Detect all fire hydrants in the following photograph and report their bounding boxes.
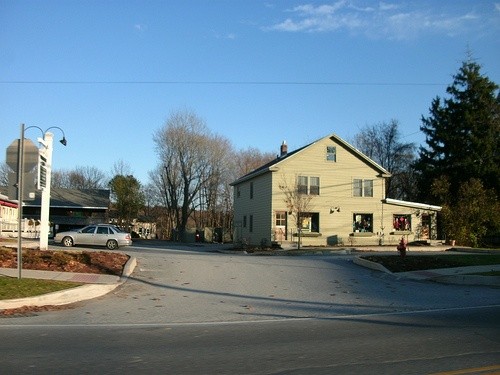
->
[397,237,406,258]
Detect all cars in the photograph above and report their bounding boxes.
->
[54,223,133,250]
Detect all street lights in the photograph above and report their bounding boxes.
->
[22,125,67,251]
